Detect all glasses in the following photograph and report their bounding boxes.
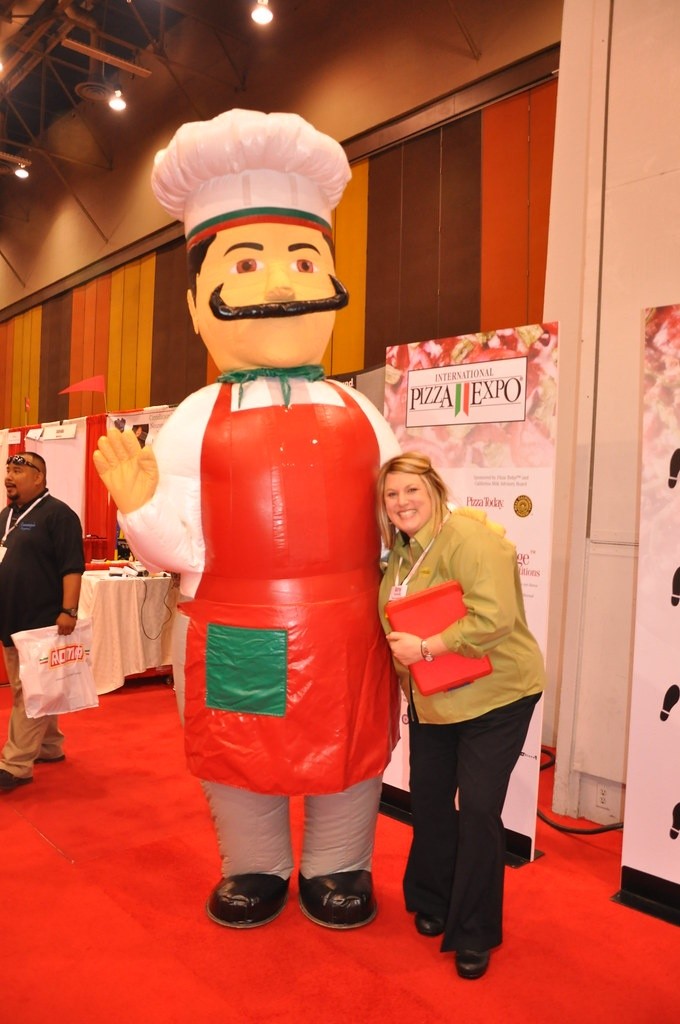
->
[6,456,41,472]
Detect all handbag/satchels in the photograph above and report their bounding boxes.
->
[10,620,99,719]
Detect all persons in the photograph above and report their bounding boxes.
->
[394,589,400,596]
[379,452,546,979]
[94,107,506,926]
[0,452,86,795]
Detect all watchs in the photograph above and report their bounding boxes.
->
[421,640,433,661]
[62,609,77,617]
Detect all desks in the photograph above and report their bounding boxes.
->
[76,570,181,697]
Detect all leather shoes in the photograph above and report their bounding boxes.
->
[456,950,489,978]
[415,914,444,936]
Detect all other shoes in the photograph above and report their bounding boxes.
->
[36,754,65,763]
[0,769,33,790]
